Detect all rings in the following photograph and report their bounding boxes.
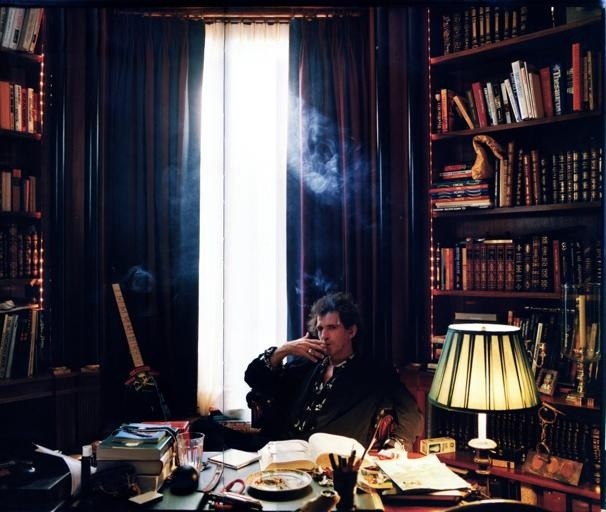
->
[309,348,314,355]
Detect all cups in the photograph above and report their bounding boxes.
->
[175,431,205,479]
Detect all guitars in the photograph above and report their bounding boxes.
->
[111,284,174,422]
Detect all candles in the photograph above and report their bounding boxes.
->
[579,295,587,348]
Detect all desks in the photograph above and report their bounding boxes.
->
[154,452,386,511]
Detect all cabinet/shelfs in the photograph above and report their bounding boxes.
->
[0,8,100,450]
[407,6,602,512]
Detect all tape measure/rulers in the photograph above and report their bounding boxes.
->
[112,283,144,369]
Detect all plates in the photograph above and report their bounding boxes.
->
[245,468,313,497]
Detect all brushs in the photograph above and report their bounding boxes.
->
[358,415,394,469]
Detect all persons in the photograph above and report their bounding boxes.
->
[244,293,423,463]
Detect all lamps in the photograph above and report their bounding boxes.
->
[428,323,540,499]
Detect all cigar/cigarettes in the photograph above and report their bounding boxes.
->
[320,344,326,348]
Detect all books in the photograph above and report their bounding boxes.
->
[255,432,369,465]
[95,418,189,493]
[376,455,472,507]
[0,3,48,379]
[209,448,257,470]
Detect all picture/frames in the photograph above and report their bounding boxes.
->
[536,369,557,398]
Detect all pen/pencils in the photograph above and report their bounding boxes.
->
[327,451,355,471]
